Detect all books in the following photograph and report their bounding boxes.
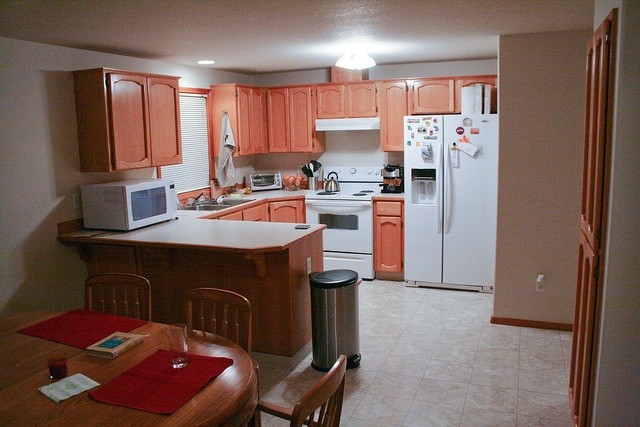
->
[84,331,145,360]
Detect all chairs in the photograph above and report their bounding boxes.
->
[81,270,153,318]
[247,353,351,427]
[182,285,253,356]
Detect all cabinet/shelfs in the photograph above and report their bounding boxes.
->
[375,200,402,272]
[74,68,152,171]
[379,80,410,152]
[313,81,376,117]
[265,86,327,155]
[209,82,252,156]
[248,84,264,156]
[147,74,182,165]
[269,200,305,223]
[413,74,499,115]
[218,202,271,224]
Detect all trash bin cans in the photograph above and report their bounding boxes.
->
[308,268,361,371]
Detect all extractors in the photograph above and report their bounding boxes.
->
[315,117,384,134]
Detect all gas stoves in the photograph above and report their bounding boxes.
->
[305,183,379,201]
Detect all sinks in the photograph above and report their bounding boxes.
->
[181,205,230,211]
[205,199,255,206]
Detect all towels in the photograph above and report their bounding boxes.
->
[88,347,236,418]
[17,308,147,351]
[215,111,236,189]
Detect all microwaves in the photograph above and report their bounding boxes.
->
[81,176,179,232]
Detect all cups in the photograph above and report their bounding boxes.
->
[164,323,190,369]
[46,348,70,379]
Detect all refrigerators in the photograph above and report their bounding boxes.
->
[402,114,499,294]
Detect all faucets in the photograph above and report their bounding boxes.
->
[192,192,204,206]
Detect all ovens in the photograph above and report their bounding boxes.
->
[244,171,283,192]
[305,199,375,281]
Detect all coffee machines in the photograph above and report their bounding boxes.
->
[378,163,404,195]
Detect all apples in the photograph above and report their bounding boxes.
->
[282,173,303,191]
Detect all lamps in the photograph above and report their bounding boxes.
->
[334,51,377,70]
